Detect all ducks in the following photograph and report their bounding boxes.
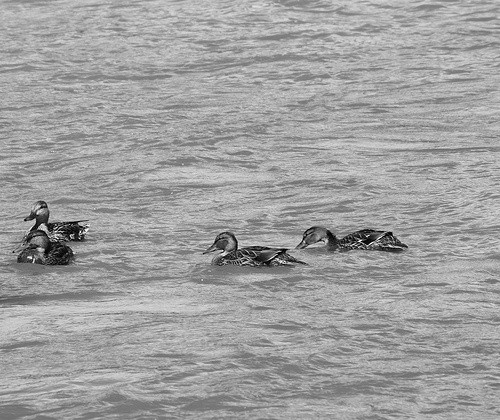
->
[202,231,311,271]
[12,201,90,265]
[294,225,409,254]
